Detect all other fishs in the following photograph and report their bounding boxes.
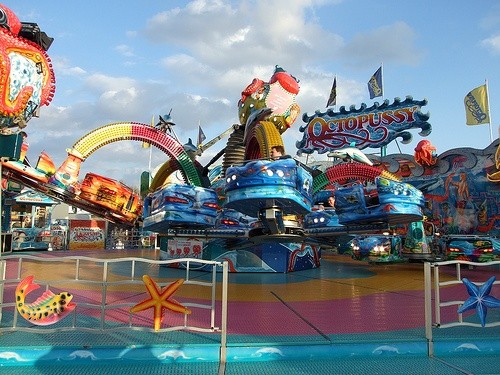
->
[16,274,77,328]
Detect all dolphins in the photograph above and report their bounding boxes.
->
[235,107,274,148]
[328,141,383,167]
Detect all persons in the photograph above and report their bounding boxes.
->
[327,196,335,207]
[271,146,284,161]
[111,227,123,235]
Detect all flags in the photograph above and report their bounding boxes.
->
[326,77,336,107]
[464,84,490,125]
[368,67,382,99]
